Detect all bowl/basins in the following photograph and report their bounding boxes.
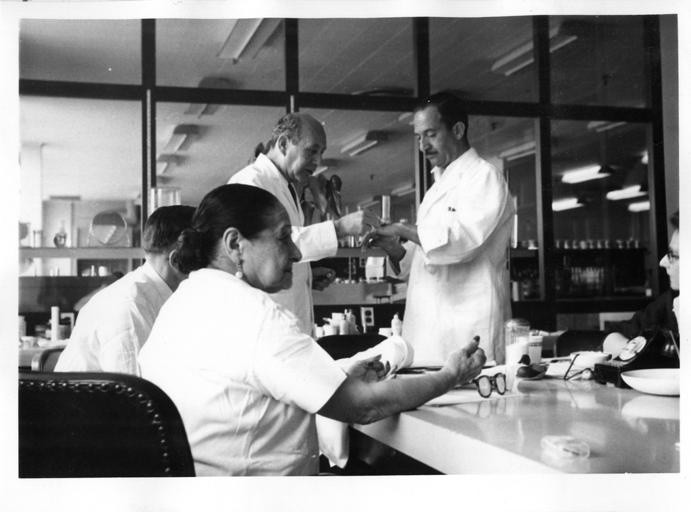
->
[571,350,606,372]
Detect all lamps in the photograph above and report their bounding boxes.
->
[339,130,387,157]
[357,141,537,211]
[490,20,579,78]
[155,20,283,184]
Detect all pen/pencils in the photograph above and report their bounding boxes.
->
[550,359,572,363]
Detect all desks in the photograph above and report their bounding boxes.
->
[347,361,680,475]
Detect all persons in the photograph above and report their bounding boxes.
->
[604,210,680,368]
[52,206,198,376]
[360,88,514,366]
[143,184,486,476]
[227,113,380,338]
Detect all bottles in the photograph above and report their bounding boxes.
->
[314,307,357,340]
[18,315,27,341]
[503,319,531,391]
[513,238,651,300]
[391,312,402,337]
[526,335,543,363]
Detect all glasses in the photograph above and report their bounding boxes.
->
[458,373,505,397]
[668,250,679,262]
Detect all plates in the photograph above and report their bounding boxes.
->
[619,367,681,396]
